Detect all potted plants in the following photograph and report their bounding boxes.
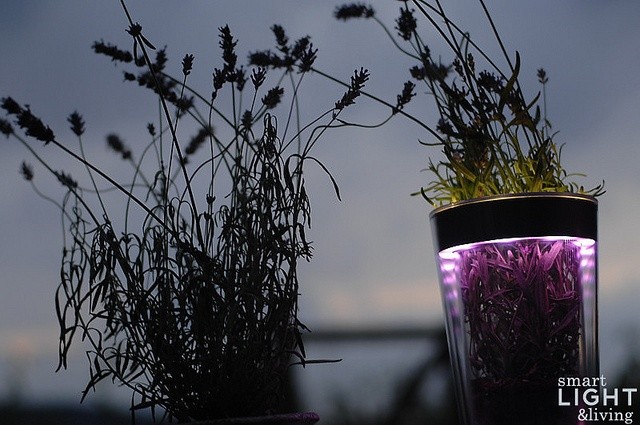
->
[247,0,606,425]
